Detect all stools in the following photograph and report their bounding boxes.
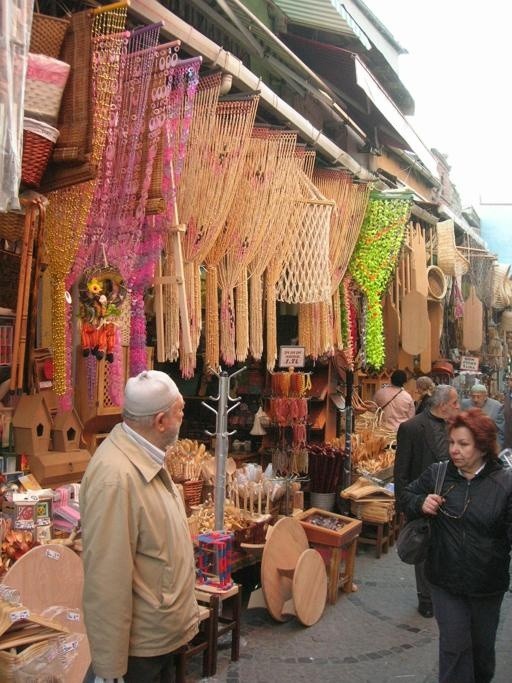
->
[171,572,244,682]
[353,509,404,559]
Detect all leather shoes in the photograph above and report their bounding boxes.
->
[418,602,433,617]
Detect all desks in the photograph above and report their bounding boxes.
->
[240,517,329,626]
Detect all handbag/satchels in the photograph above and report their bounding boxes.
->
[396,519,431,564]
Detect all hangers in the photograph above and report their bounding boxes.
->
[0,583,70,652]
[47,483,83,536]
[340,470,397,524]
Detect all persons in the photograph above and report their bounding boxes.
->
[78,369,201,683]
[375,370,512,453]
[393,385,460,618]
[399,408,512,683]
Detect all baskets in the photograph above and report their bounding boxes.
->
[184,481,205,517]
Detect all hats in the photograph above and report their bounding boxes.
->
[123,370,178,416]
[433,361,452,374]
